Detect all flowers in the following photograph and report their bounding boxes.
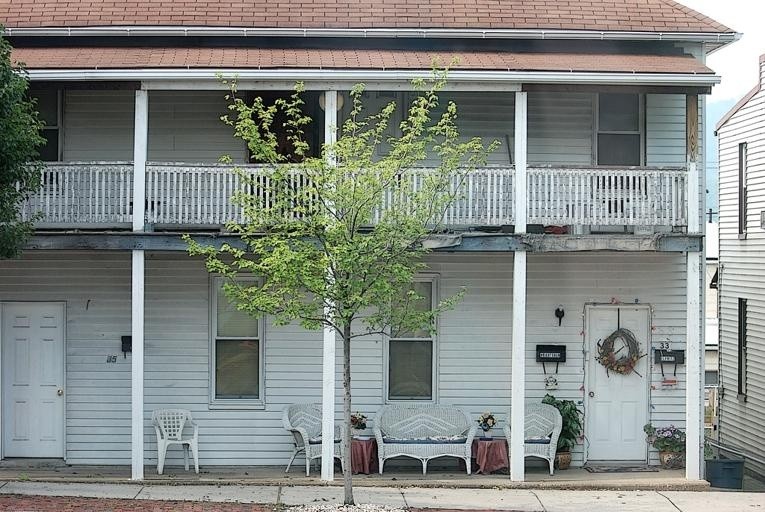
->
[642,421,684,453]
[350,411,367,429]
[594,329,647,379]
[477,411,497,429]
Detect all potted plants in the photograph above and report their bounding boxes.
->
[542,394,584,469]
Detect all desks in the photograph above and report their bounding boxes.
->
[348,438,375,474]
[474,438,508,473]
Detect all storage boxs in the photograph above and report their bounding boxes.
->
[704,457,745,489]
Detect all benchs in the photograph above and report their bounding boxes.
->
[372,403,478,474]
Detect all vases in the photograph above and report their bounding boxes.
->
[659,454,684,467]
[352,430,362,436]
[482,430,492,437]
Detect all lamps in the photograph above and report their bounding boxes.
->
[555,302,567,326]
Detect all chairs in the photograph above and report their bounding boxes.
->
[284,400,344,477]
[151,407,203,477]
[503,402,564,468]
